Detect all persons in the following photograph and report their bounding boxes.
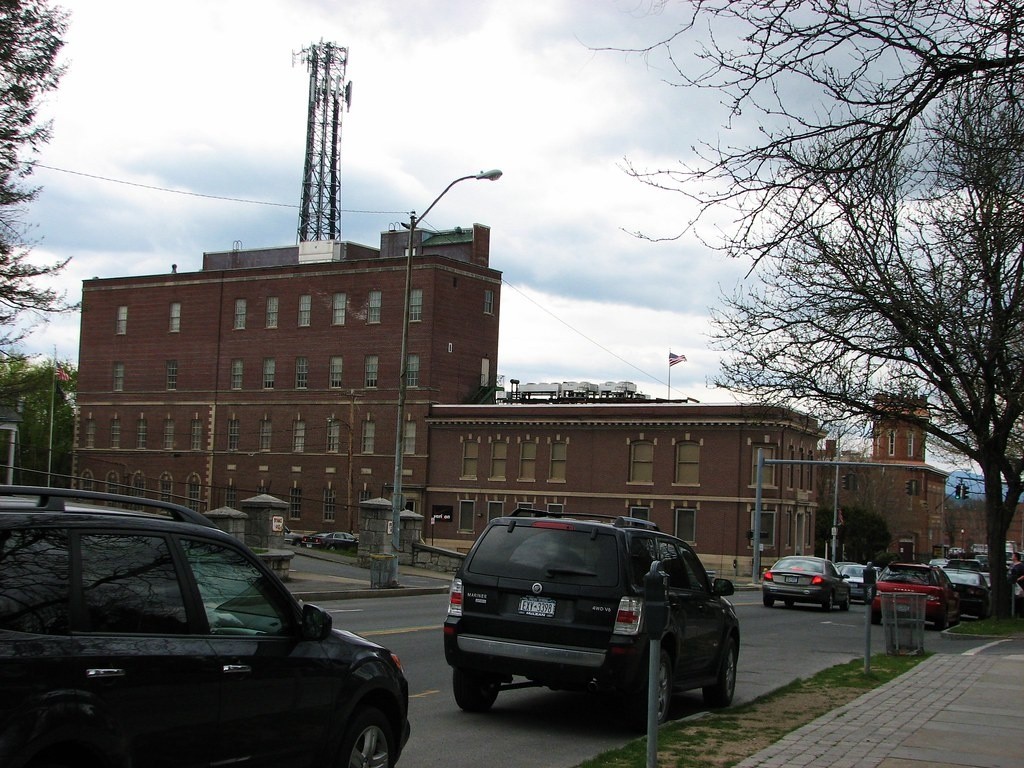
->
[863,562,876,602]
[1007,552,1024,621]
[128,555,244,628]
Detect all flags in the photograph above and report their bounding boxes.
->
[669,353,688,367]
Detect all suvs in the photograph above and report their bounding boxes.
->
[443,508,739,726]
[871,561,961,630]
[0,485,412,768]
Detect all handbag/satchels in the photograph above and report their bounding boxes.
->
[1015,582,1024,597]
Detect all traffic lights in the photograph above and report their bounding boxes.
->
[962,485,969,500]
[915,481,920,495]
[841,474,848,490]
[954,485,961,499]
[905,480,912,495]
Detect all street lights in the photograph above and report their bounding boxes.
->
[961,529,965,552]
[391,168,505,587]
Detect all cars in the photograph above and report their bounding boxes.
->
[928,540,1024,620]
[834,562,862,573]
[839,565,882,601]
[284,525,305,547]
[302,532,359,552]
[761,555,851,612]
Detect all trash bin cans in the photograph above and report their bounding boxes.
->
[877,590,927,657]
[369,552,400,590]
